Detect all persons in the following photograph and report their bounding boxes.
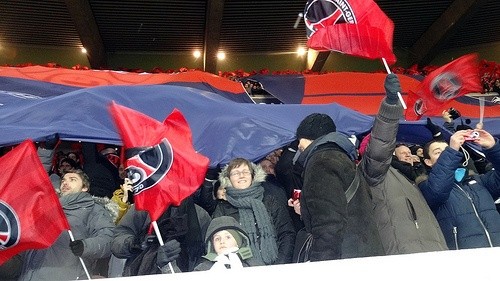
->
[0,69,500,281]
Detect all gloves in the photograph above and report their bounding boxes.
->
[131,233,157,251]
[383,74,402,105]
[155,239,182,268]
[69,239,84,257]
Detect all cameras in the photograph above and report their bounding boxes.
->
[292,189,302,204]
[464,130,480,140]
[448,107,461,120]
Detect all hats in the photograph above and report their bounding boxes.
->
[295,113,336,141]
[211,179,227,200]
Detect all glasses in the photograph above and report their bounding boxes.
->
[229,171,252,176]
[395,143,409,148]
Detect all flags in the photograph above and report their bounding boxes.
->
[403,51,482,122]
[108,99,210,234]
[0,139,71,265]
[303,0,397,67]
